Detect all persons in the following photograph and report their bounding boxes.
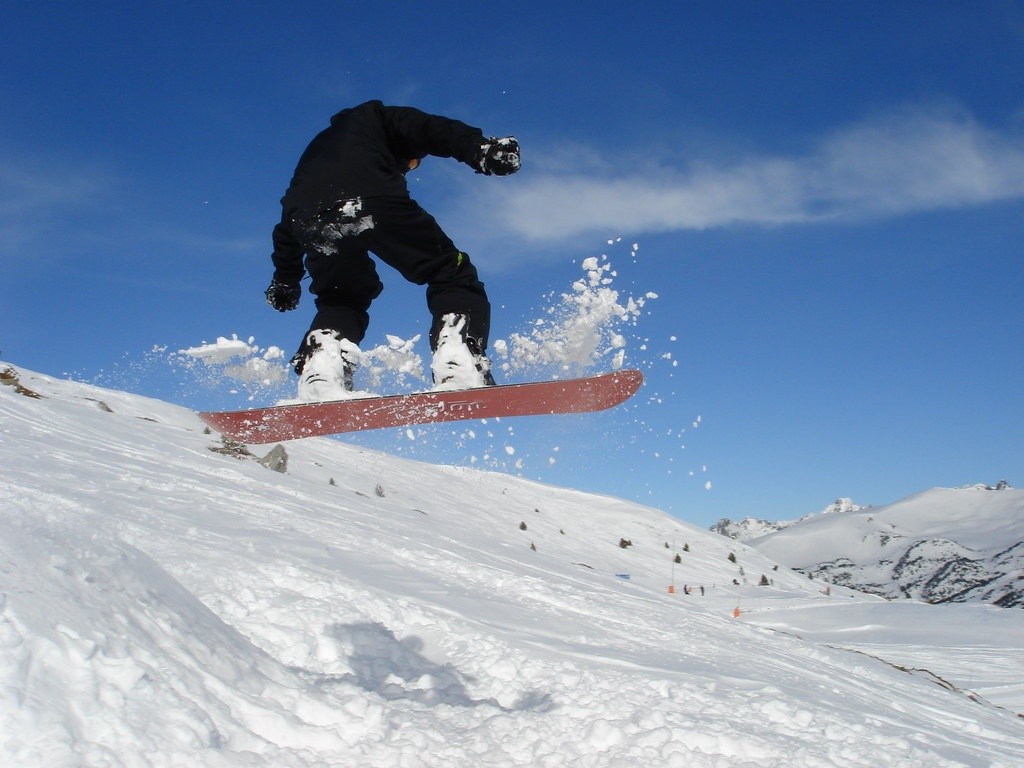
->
[265,100,521,401]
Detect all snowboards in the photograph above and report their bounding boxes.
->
[193,368,643,445]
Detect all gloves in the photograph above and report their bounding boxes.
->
[265,268,306,312]
[474,136,522,176]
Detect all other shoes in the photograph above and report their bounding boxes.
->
[431,311,497,385]
[298,328,359,395]
